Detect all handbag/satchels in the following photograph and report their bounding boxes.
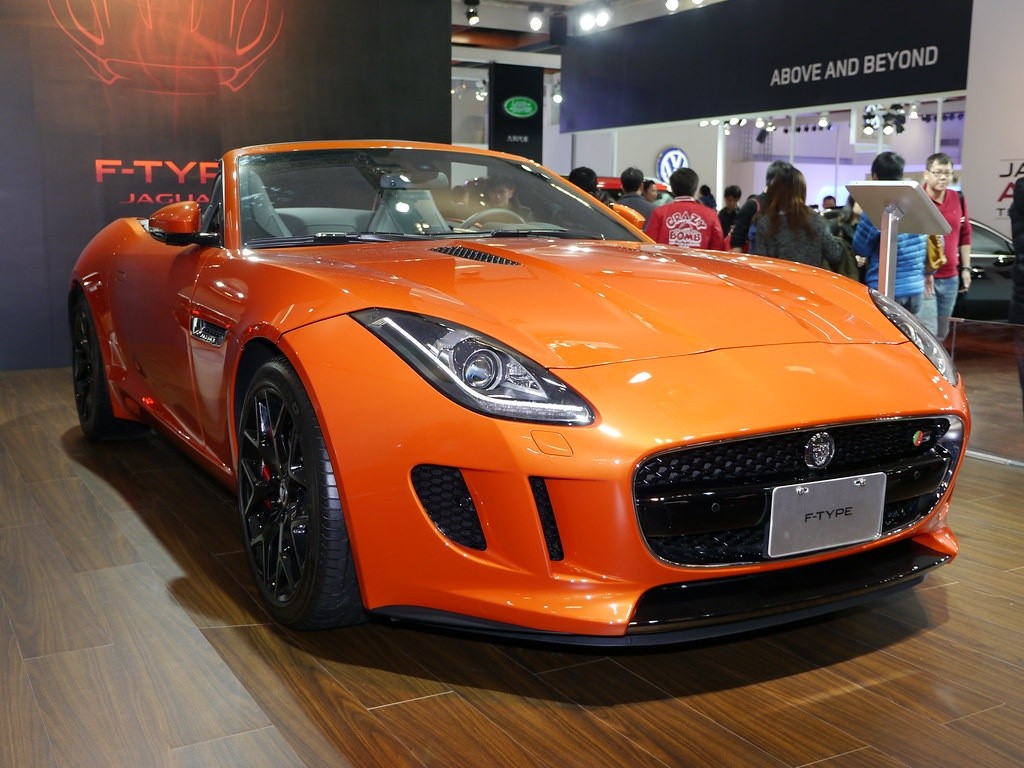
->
[723,227,751,254]
[925,232,948,275]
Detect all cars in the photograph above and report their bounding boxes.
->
[954,219,1018,332]
[561,174,677,237]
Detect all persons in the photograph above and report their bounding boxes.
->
[718,161,866,285]
[922,152,972,340]
[477,172,534,223]
[619,167,656,228]
[696,185,717,212]
[645,167,724,250]
[642,180,657,201]
[852,151,934,315]
[569,167,614,205]
[1010,176,1024,324]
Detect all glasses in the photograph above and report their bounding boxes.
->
[925,168,953,177]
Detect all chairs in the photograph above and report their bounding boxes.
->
[367,189,451,234]
[200,166,293,245]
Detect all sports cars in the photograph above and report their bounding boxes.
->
[64,138,973,648]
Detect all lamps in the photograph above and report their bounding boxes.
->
[551,90,563,104]
[766,117,776,132]
[756,129,768,144]
[907,103,920,120]
[755,117,765,128]
[783,124,833,134]
[817,112,829,127]
[465,6,479,26]
[893,115,906,134]
[862,114,876,135]
[882,113,895,135]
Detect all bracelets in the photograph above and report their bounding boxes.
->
[962,267,971,271]
